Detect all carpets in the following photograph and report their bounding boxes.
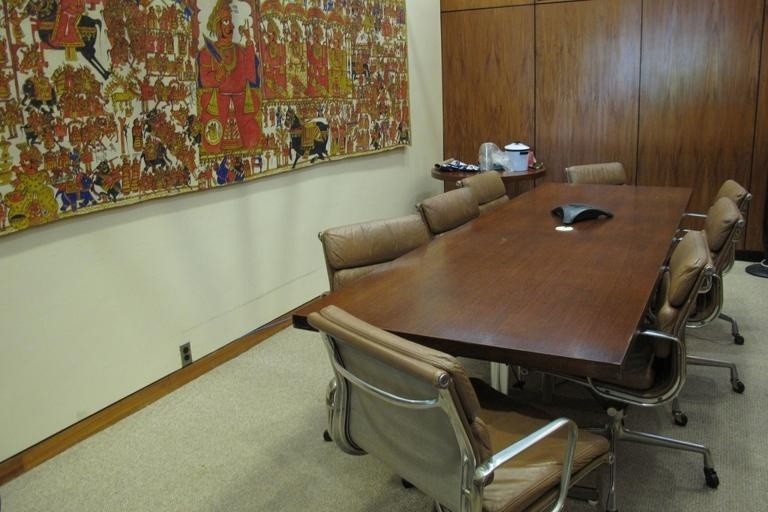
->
[0,260,768,512]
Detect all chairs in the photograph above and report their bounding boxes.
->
[536,230,720,512]
[415,187,481,238]
[565,162,626,184]
[574,197,746,427]
[456,170,510,212]
[307,304,617,512]
[317,214,434,293]
[676,180,752,345]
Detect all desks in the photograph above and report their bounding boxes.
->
[432,164,549,204]
[291,182,693,396]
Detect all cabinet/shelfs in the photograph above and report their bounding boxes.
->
[440,1,768,253]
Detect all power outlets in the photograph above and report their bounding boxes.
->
[180,342,192,368]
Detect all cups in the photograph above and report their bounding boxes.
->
[481,143,494,172]
[504,141,530,172]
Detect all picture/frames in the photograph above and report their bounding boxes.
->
[0,0,411,235]
